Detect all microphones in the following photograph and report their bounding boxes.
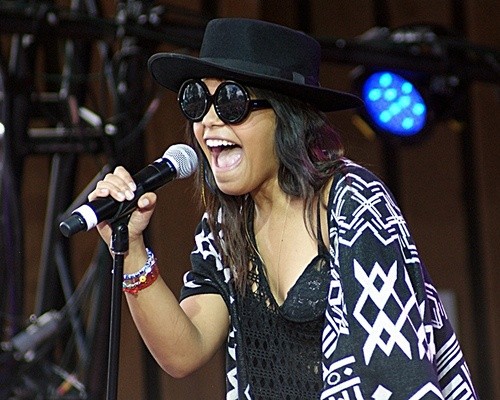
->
[58,143,199,238]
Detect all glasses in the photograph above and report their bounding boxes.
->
[177,79,273,125]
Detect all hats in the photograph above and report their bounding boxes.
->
[147,17,364,112]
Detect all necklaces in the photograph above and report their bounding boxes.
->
[243,193,291,302]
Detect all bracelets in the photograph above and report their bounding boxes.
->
[112,249,160,293]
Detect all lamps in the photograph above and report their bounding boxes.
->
[351,33,460,138]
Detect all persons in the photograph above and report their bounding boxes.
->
[88,20,478,399]
